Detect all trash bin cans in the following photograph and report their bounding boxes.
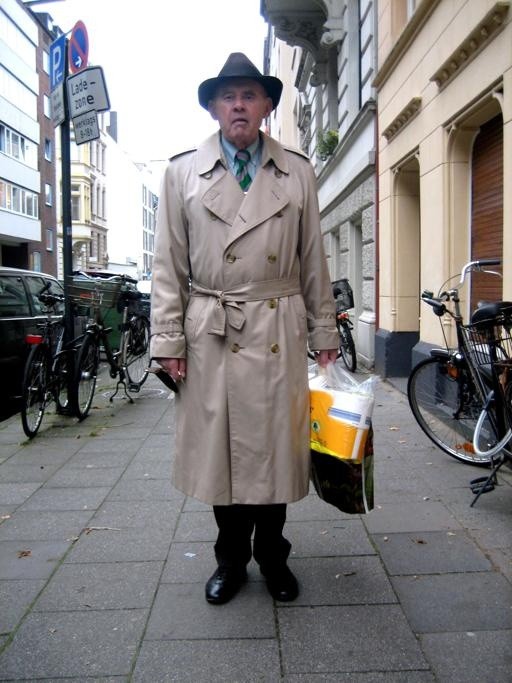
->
[60,279,125,352]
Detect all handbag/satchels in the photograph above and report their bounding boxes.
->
[298,358,377,514]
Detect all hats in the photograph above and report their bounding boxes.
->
[197,51,282,113]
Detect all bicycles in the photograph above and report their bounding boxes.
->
[406,260,512,508]
[308,278,357,372]
[17,271,152,436]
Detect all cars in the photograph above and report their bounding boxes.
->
[0,266,100,414]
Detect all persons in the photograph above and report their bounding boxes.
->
[149,51,343,604]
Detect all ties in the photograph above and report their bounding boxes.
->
[232,151,253,192]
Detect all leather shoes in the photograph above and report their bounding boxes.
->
[258,562,301,602]
[204,564,250,606]
